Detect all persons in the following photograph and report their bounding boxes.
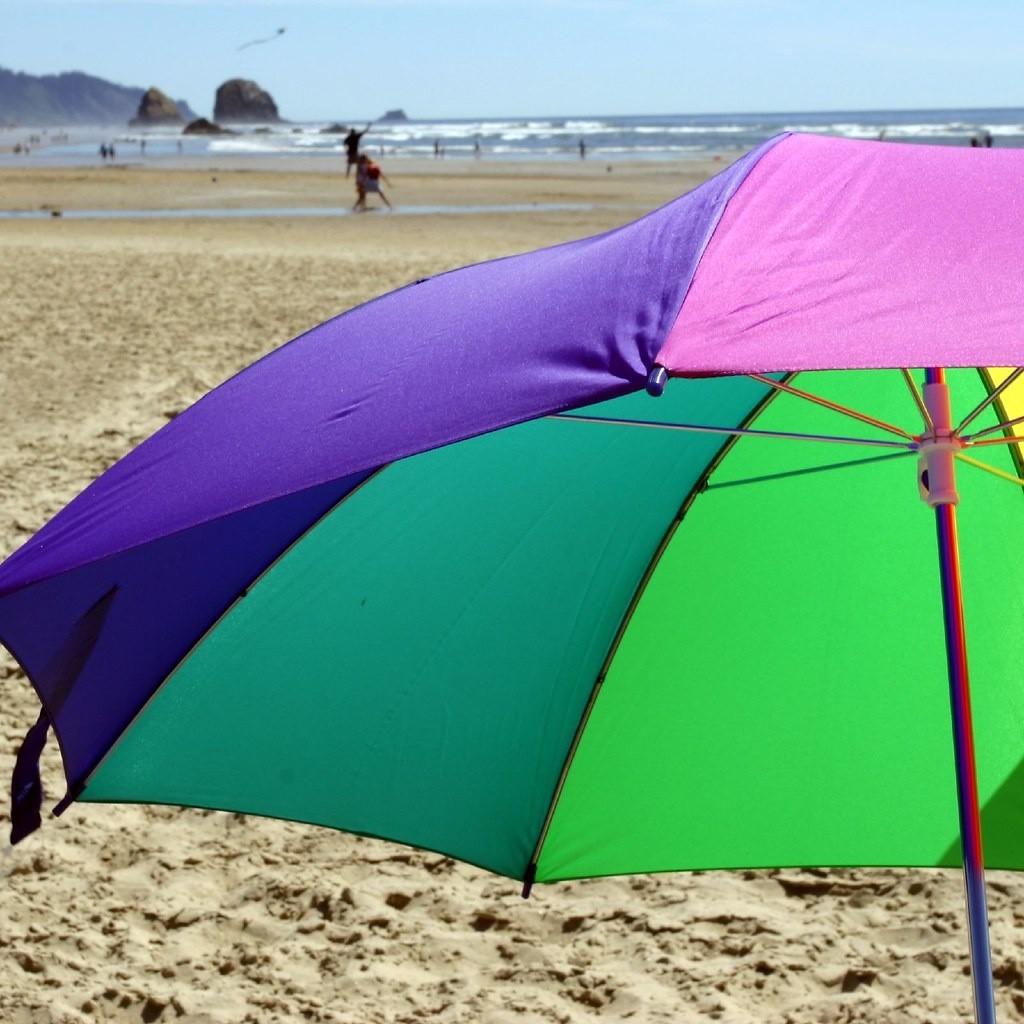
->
[579,139,585,159]
[99,144,116,159]
[344,123,394,210]
[434,138,438,152]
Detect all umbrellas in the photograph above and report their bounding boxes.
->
[0,131,1024,1023]
